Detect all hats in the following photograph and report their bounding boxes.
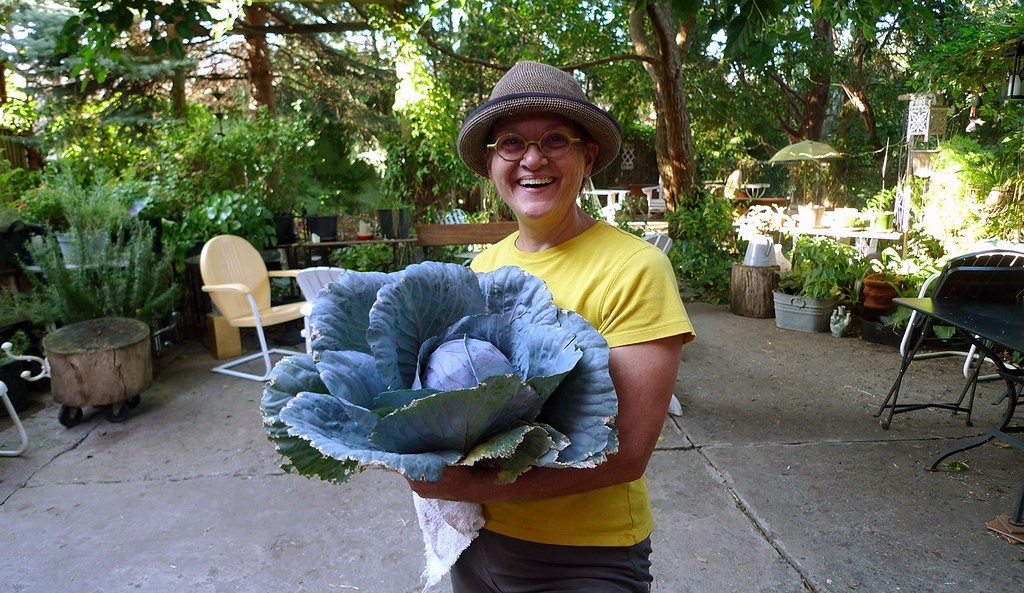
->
[458,61,623,180]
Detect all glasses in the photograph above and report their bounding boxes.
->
[485,130,582,162]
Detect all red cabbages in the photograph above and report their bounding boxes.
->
[259,257,622,486]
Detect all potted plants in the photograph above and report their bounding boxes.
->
[870,208,894,232]
[798,201,825,227]
[834,205,858,226]
[180,189,277,358]
[772,235,857,332]
[851,244,908,308]
[356,211,374,239]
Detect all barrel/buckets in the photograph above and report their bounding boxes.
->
[744,234,778,268]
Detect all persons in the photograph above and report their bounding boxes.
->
[445,56,701,593]
[723,158,749,199]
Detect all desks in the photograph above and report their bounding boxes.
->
[278,236,417,297]
[741,181,770,198]
[892,297,1024,471]
[43,318,155,427]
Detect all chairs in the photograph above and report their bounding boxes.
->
[705,167,743,198]
[582,176,631,221]
[642,175,667,219]
[200,233,307,381]
[0,380,29,456]
[644,232,684,416]
[871,248,1024,429]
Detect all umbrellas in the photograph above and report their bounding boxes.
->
[767,138,843,205]
